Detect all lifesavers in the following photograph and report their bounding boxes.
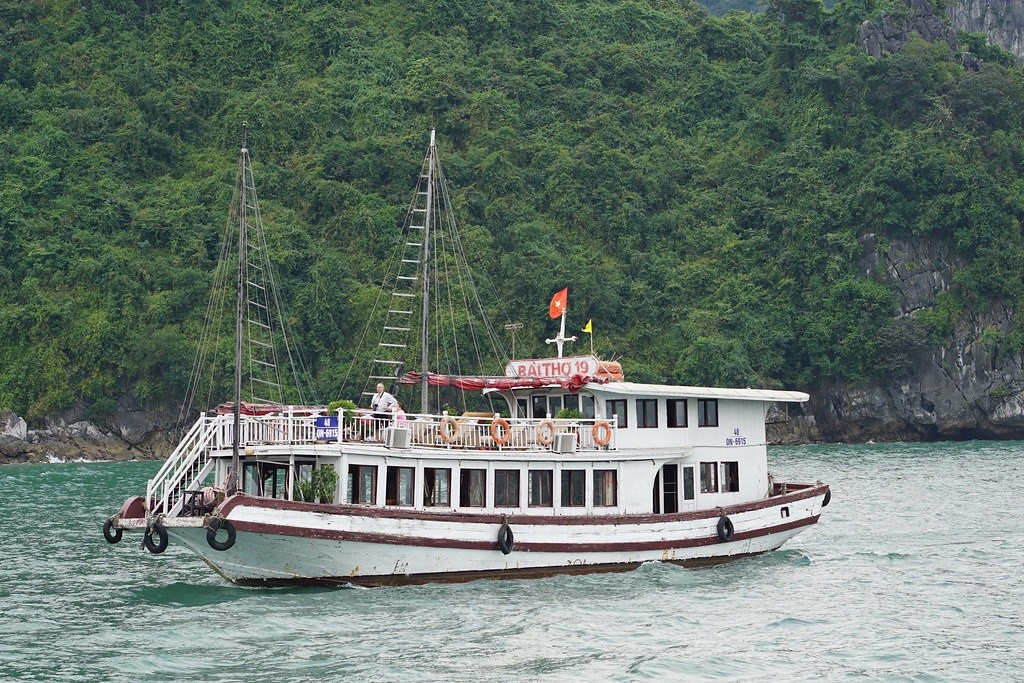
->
[206,517,237,552]
[821,486,832,507]
[536,419,557,446]
[716,516,735,542]
[592,419,612,446]
[490,417,510,444]
[144,524,169,554]
[101,517,123,545]
[497,523,514,556]
[439,416,460,444]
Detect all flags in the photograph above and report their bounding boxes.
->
[549,286,567,319]
[581,319,592,334]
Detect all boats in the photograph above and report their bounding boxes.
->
[103,120,834,592]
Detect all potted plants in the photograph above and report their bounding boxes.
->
[328,399,359,441]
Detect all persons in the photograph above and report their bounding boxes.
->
[371,383,406,441]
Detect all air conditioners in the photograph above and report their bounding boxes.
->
[385,426,411,448]
[553,433,578,454]
